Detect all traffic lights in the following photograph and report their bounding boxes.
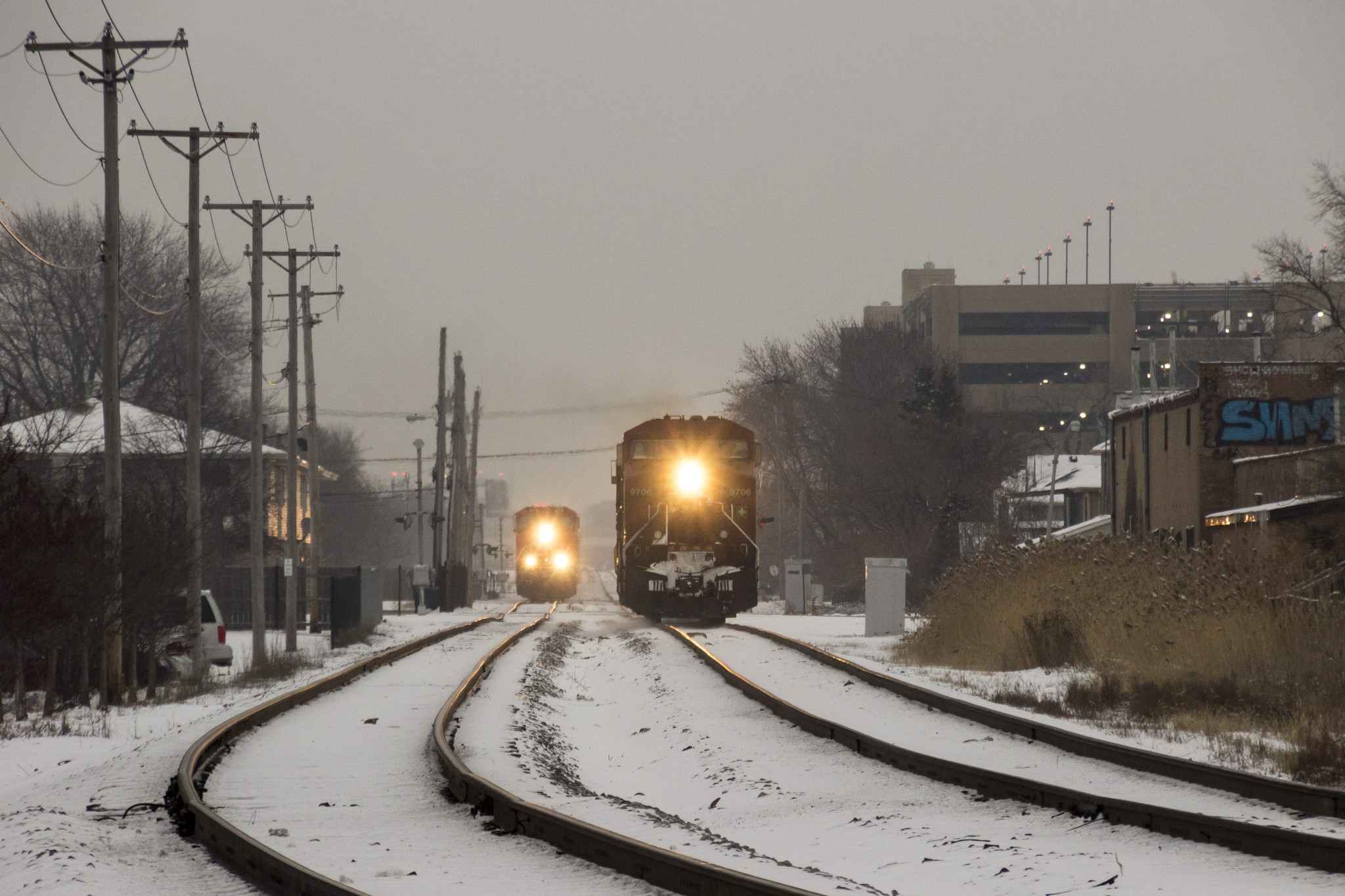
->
[394,513,412,531]
[430,511,445,530]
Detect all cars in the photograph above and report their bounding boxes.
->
[134,587,234,688]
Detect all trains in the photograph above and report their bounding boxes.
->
[512,505,582,603]
[611,414,762,628]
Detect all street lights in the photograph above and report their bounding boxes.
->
[406,414,460,564]
